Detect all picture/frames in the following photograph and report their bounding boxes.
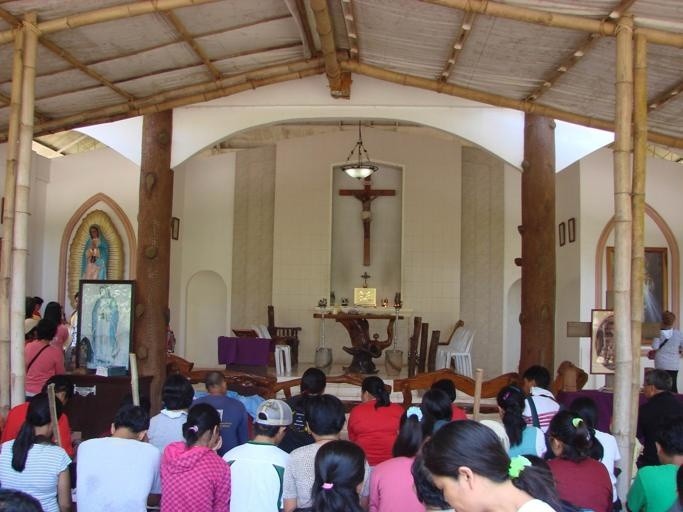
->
[605,247,669,339]
[75,279,137,374]
[589,308,617,375]
[172,216,181,242]
[568,217,576,244]
[558,221,566,246]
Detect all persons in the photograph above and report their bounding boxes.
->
[150,377,222,458]
[421,422,559,512]
[349,380,404,464]
[289,368,326,449]
[222,399,290,512]
[418,390,451,442]
[520,366,559,428]
[624,418,683,512]
[161,404,231,511]
[636,372,683,444]
[1,376,72,461]
[293,440,370,511]
[567,398,624,511]
[91,286,119,371]
[0,489,44,512]
[485,387,548,460]
[432,379,468,422]
[509,448,593,512]
[79,224,109,278]
[651,312,681,392]
[37,302,68,350]
[25,319,64,396]
[76,407,161,511]
[192,372,248,451]
[25,297,40,334]
[282,395,375,511]
[0,395,73,512]
[539,413,612,511]
[370,406,440,511]
[65,294,79,369]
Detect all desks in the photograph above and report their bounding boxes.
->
[313,308,404,376]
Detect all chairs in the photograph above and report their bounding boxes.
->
[436,321,476,376]
[252,299,301,374]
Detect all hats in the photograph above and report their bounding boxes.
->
[253,397,293,427]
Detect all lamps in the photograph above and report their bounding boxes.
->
[342,118,380,180]
